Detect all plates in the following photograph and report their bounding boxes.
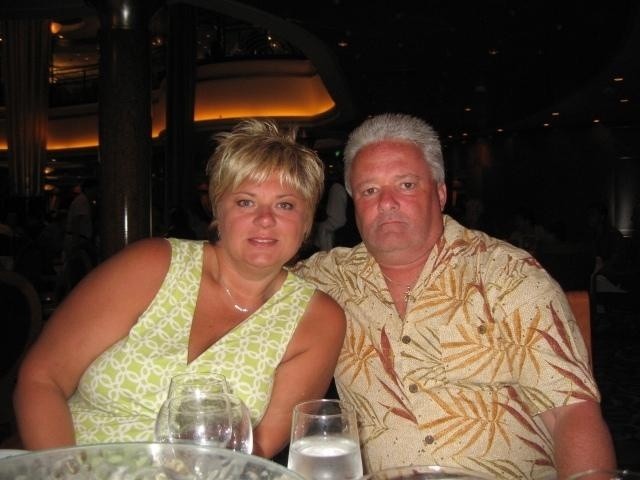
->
[0,442,304,480]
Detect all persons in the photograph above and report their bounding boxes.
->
[286,114,619,480]
[11,119,347,460]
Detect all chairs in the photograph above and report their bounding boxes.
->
[561,294,592,377]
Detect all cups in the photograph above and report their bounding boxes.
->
[151,391,253,480]
[164,371,235,480]
[286,399,363,480]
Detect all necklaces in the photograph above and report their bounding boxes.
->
[220,276,278,314]
[379,267,429,304]
